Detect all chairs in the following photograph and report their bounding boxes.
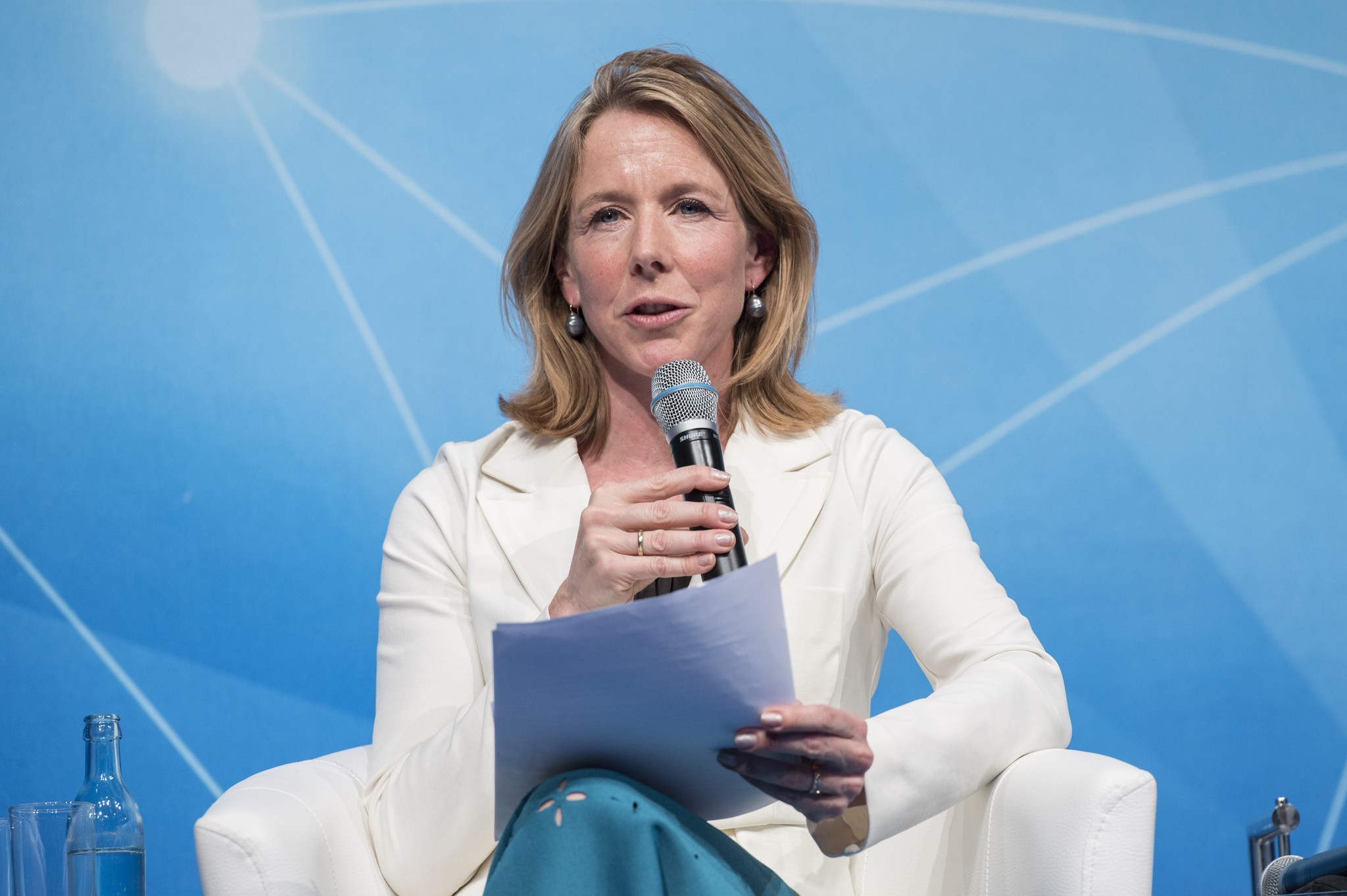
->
[196,748,1159,896]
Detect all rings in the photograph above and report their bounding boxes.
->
[638,530,643,556]
[810,763,820,794]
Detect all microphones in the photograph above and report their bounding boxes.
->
[649,360,748,582]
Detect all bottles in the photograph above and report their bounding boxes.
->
[64,713,145,896]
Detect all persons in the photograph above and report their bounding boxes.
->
[367,49,1072,896]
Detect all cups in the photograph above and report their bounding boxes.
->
[0,801,95,896]
